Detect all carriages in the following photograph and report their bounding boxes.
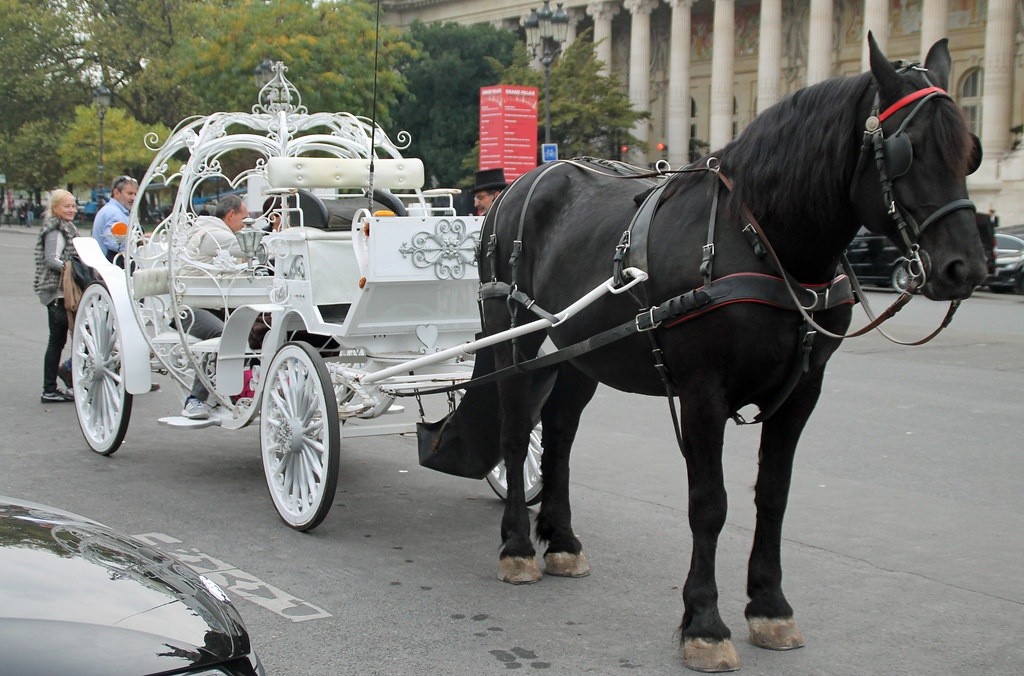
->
[57,31,1002,676]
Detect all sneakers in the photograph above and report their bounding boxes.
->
[181,397,210,419]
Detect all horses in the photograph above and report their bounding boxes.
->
[477,25,992,672]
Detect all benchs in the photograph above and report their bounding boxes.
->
[265,156,462,243]
[135,267,203,344]
[176,276,342,356]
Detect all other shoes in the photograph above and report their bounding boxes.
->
[151,383,160,391]
[65,388,93,403]
[41,389,76,402]
[57,366,73,389]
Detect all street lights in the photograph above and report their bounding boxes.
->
[92,86,111,209]
[526,1,570,164]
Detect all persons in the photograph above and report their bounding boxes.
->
[59,174,160,391]
[167,194,340,420]
[16,195,29,226]
[33,189,98,403]
[2,191,13,227]
[471,167,508,214]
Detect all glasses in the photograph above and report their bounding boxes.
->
[474,190,494,202]
[114,175,137,187]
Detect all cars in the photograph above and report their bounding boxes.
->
[0,491,269,676]
[843,220,1024,294]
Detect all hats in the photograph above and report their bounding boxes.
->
[469,168,508,195]
